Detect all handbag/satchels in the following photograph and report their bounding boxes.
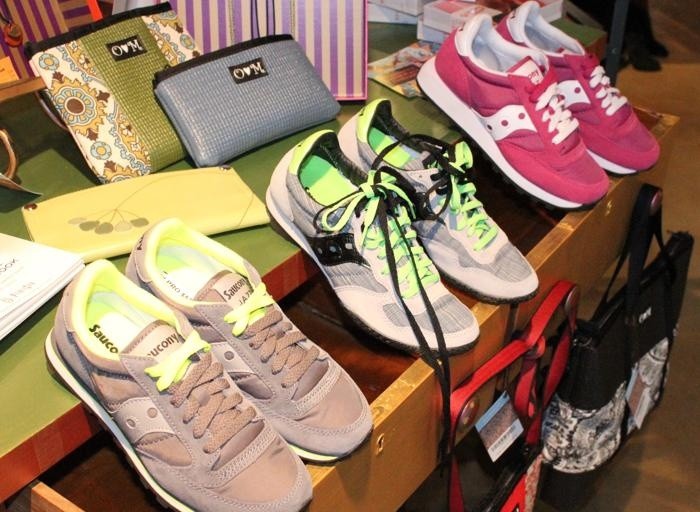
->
[542,231,692,511]
[22,165,271,264]
[23,3,205,184]
[154,34,342,168]
[478,447,543,511]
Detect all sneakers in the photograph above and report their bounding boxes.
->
[336,99,539,303]
[417,14,610,208]
[125,218,374,463]
[45,259,315,512]
[496,1,660,175]
[265,131,481,355]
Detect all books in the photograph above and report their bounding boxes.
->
[0,232,85,341]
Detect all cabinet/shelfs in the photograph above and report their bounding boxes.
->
[1,21,682,512]
